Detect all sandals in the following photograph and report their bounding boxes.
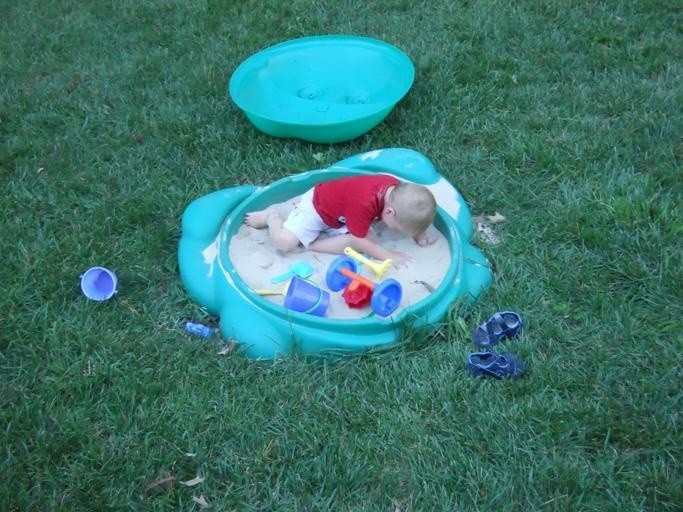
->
[467,347,525,381]
[473,223,500,250]
[473,311,522,347]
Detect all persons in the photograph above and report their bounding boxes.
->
[243,174,440,270]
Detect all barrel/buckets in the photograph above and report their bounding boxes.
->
[284,274,330,316]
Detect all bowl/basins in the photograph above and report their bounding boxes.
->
[227,34,420,140]
